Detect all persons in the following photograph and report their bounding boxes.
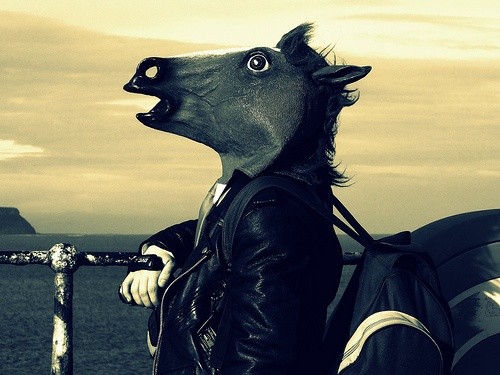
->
[115,18,373,373]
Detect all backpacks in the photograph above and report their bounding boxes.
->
[225,172,459,375]
